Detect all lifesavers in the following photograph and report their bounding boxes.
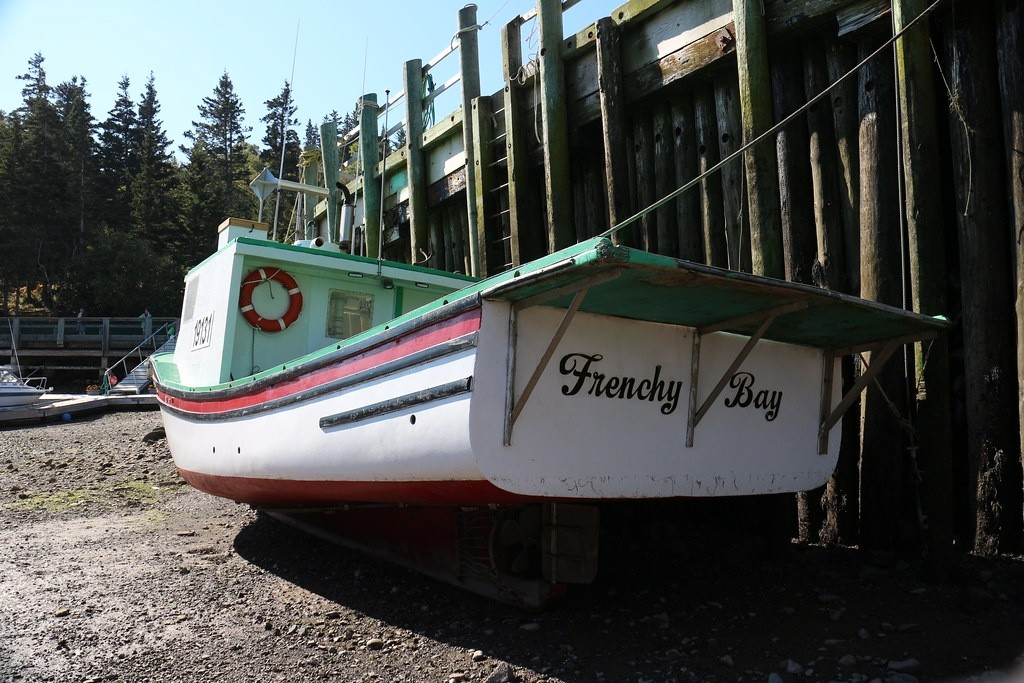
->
[237,264,305,334]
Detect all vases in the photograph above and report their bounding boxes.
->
[86,390,105,395]
[148,389,157,394]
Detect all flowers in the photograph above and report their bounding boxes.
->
[149,384,156,389]
[87,385,101,390]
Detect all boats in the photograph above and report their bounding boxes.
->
[148,218,954,609]
[0,365,49,407]
